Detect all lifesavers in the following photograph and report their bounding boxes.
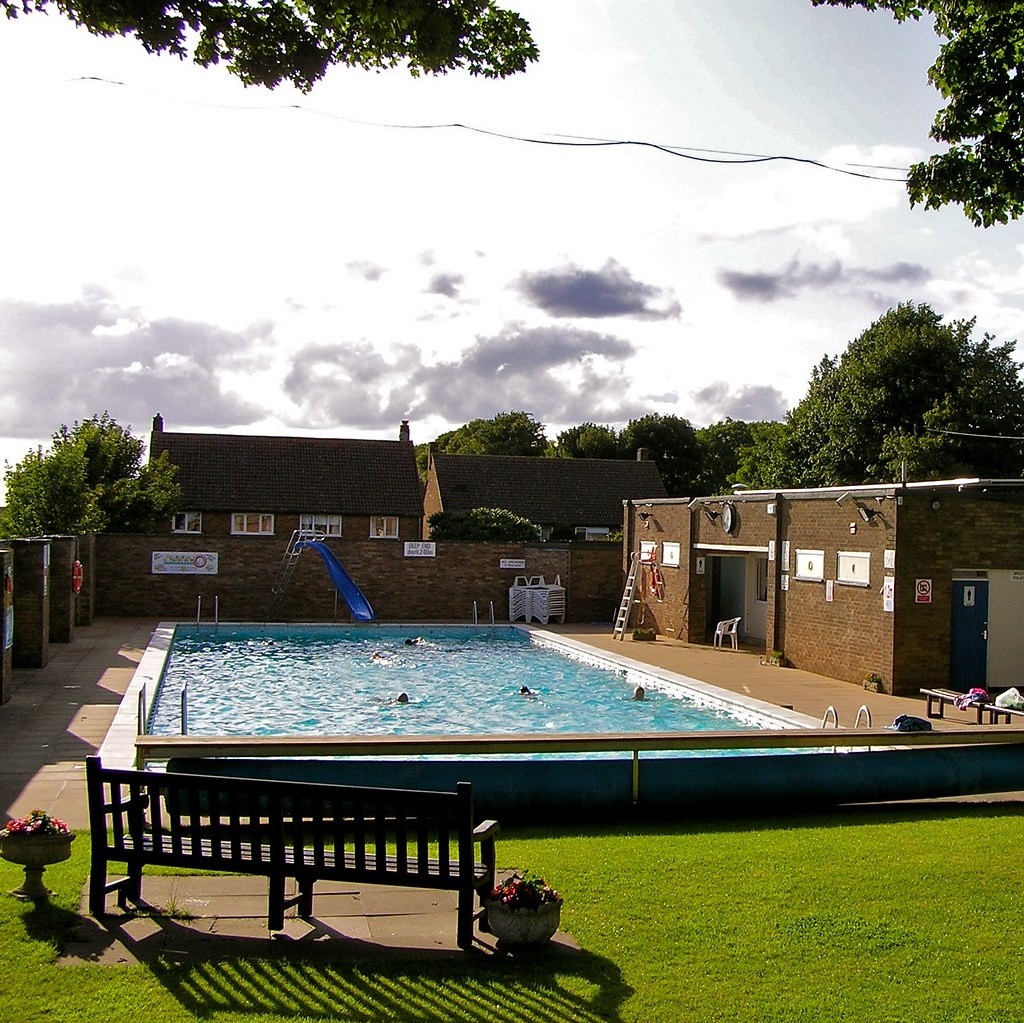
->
[73,559,84,591]
[649,567,660,596]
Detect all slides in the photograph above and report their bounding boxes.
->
[294,539,376,622]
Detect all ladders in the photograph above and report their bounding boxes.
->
[613,551,678,641]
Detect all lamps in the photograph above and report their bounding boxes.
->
[857,506,882,521]
[638,512,653,520]
[704,511,721,520]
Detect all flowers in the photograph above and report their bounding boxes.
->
[490,868,562,910]
[6,806,66,834]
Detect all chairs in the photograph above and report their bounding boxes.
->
[714,617,742,649]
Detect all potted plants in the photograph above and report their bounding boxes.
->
[863,674,882,693]
[632,628,656,641]
[770,651,789,666]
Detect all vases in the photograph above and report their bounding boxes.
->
[0,829,76,901]
[485,896,563,945]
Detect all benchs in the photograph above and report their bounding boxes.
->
[920,688,1024,725]
[88,756,497,947]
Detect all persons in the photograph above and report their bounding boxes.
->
[372,652,380,658]
[405,637,423,645]
[632,686,645,700]
[398,693,408,702]
[520,686,531,694]
